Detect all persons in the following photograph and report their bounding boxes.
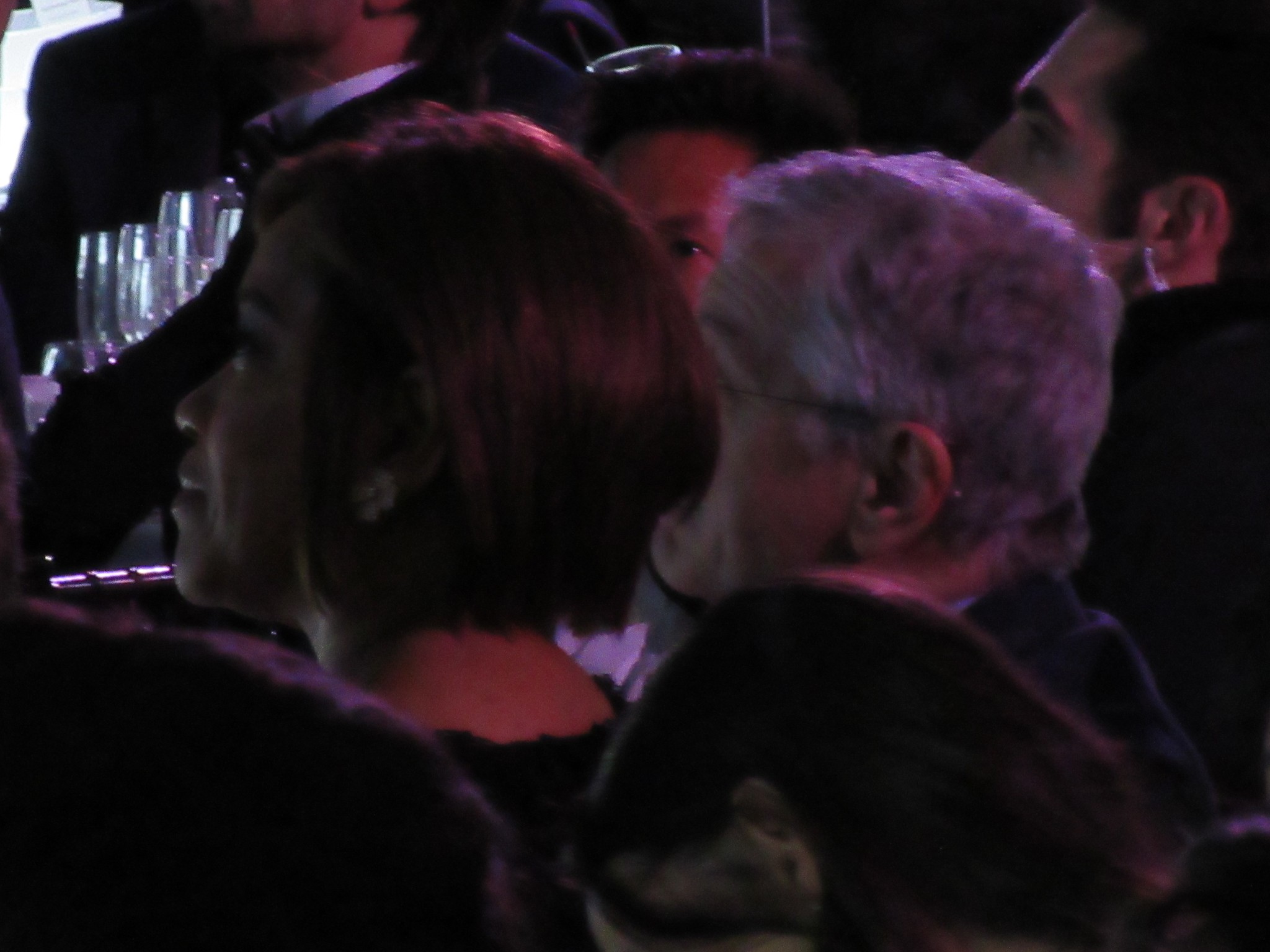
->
[955,0,1267,830]
[170,120,720,951]
[2,3,591,394]
[7,1,513,589]
[576,47,856,326]
[559,572,1269,952]
[0,579,528,951]
[659,142,1223,844]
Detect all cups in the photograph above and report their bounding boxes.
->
[45,190,247,387]
